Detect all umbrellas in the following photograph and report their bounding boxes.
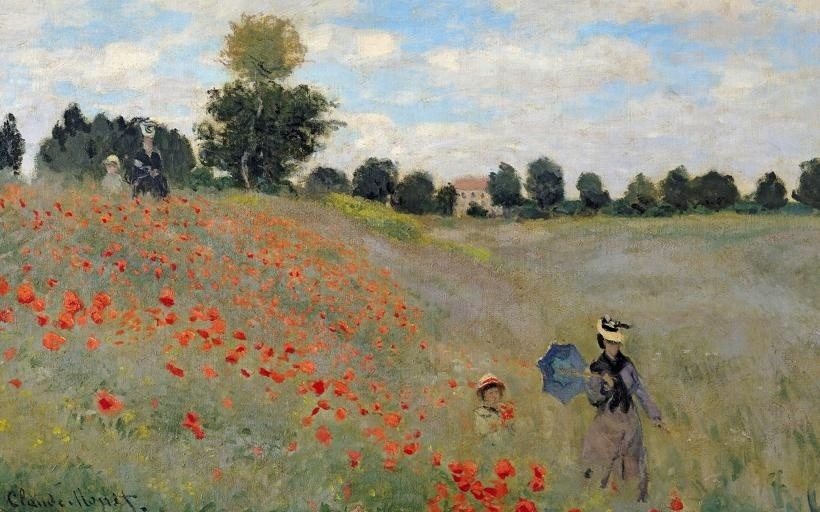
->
[535,341,617,406]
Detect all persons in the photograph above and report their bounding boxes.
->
[472,373,513,459]
[579,316,671,504]
[100,155,123,194]
[130,121,171,201]
